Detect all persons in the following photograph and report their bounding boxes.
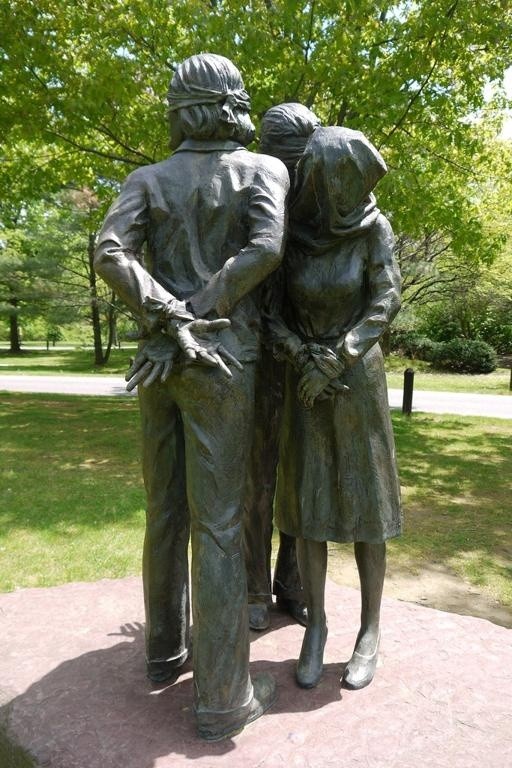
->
[93,51,289,740]
[246,102,322,630]
[254,125,403,692]
